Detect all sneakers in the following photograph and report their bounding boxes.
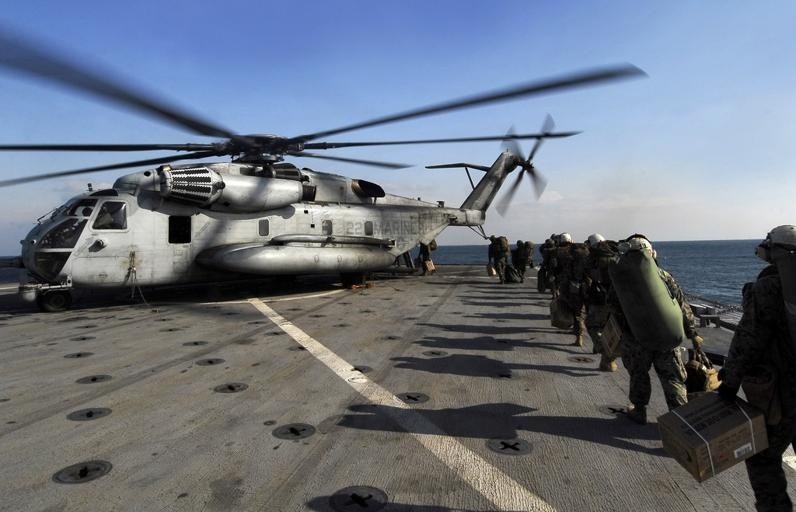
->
[575,335,583,346]
[599,359,618,372]
[629,405,648,425]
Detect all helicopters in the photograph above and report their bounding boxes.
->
[0,29,650,312]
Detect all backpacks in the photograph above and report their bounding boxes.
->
[493,236,509,255]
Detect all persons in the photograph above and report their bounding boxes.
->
[718,225,796,512]
[539,232,704,425]
[488,235,526,284]
[82,203,114,229]
[419,243,433,276]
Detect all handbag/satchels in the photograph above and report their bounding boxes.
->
[486,263,498,276]
[685,346,722,394]
[504,263,524,283]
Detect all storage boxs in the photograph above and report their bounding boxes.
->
[656,387,770,482]
[549,296,574,329]
[601,315,632,359]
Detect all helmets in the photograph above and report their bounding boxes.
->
[618,237,656,259]
[767,224,796,245]
[559,232,572,243]
[588,233,604,246]
